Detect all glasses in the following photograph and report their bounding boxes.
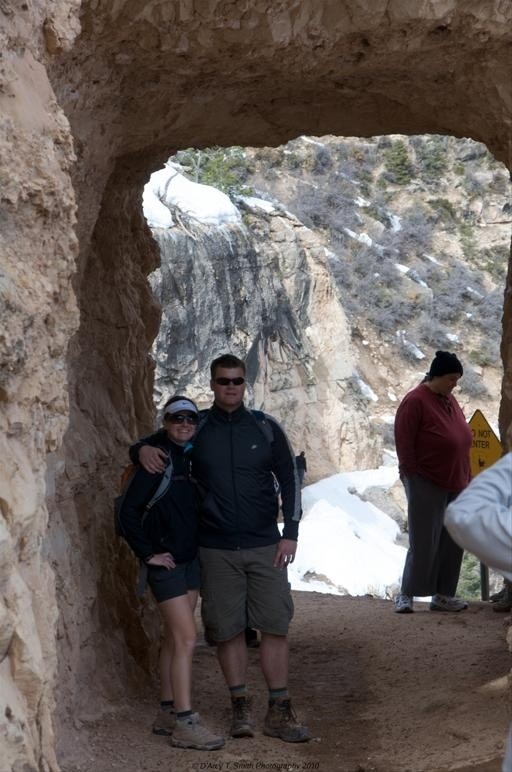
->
[214,377,245,386]
[165,415,199,425]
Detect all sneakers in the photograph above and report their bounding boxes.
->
[229,693,257,737]
[489,582,512,612]
[261,696,313,742]
[393,590,414,613]
[429,593,468,612]
[170,711,226,750]
[151,708,178,736]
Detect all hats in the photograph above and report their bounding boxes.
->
[160,399,199,422]
[428,350,463,377]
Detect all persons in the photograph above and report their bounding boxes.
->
[117,393,225,751]
[386,348,475,612]
[295,450,306,484]
[443,448,512,592]
[489,577,512,614]
[124,349,315,741]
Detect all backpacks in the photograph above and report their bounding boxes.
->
[114,462,136,538]
